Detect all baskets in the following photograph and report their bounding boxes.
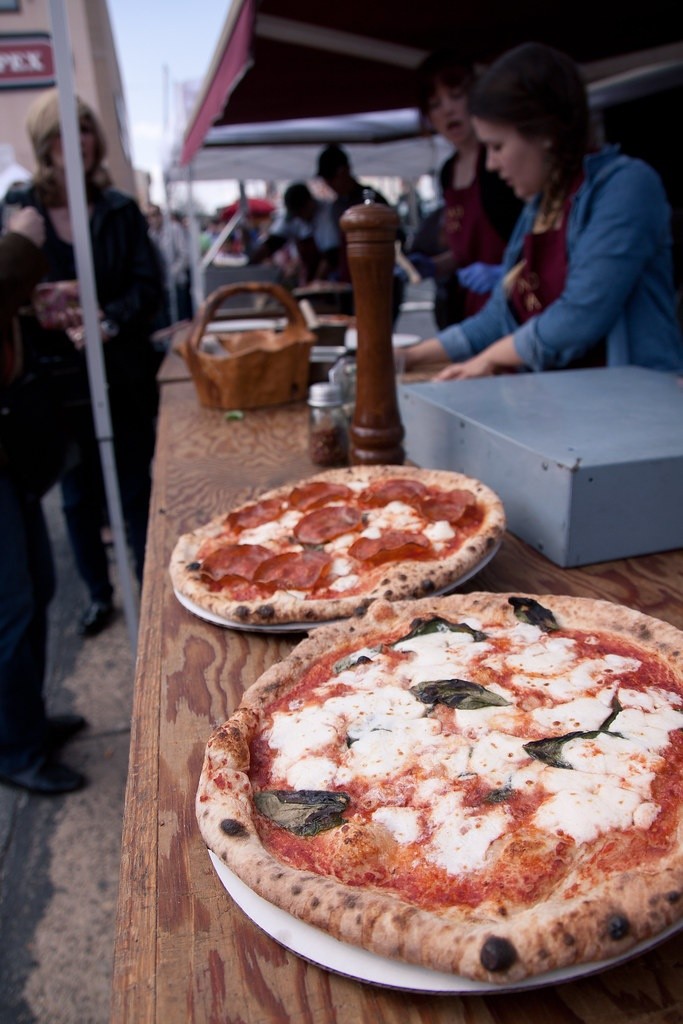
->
[172,282,316,412]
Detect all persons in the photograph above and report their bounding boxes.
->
[396,50,525,323]
[404,43,683,385]
[0,92,165,795]
[146,205,188,321]
[174,147,422,314]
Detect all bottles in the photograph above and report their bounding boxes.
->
[325,328,358,419]
[308,382,350,465]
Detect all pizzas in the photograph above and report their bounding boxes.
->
[196,590,683,983]
[169,465,505,624]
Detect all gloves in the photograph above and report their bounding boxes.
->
[459,264,503,296]
[395,253,434,285]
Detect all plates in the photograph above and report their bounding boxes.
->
[201,846,683,998]
[168,523,506,639]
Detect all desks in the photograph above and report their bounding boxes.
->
[156,326,193,382]
[109,381,682,1024]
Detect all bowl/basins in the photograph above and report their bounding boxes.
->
[392,332,424,373]
[310,345,346,383]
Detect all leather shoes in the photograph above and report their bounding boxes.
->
[6,753,89,795]
[46,714,85,741]
[75,601,115,634]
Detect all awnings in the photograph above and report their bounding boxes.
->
[180,0,683,161]
